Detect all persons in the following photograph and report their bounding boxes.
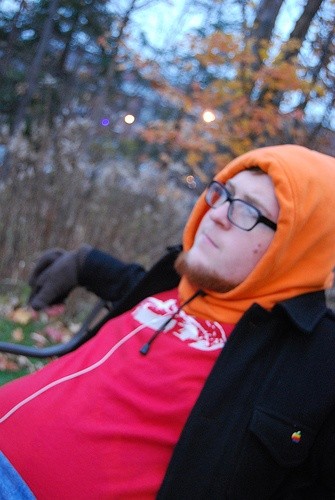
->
[0,144,335,500]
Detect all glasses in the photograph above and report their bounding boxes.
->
[205,181,277,236]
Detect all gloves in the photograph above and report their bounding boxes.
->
[28,245,91,311]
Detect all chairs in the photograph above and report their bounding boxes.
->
[0,296,116,359]
